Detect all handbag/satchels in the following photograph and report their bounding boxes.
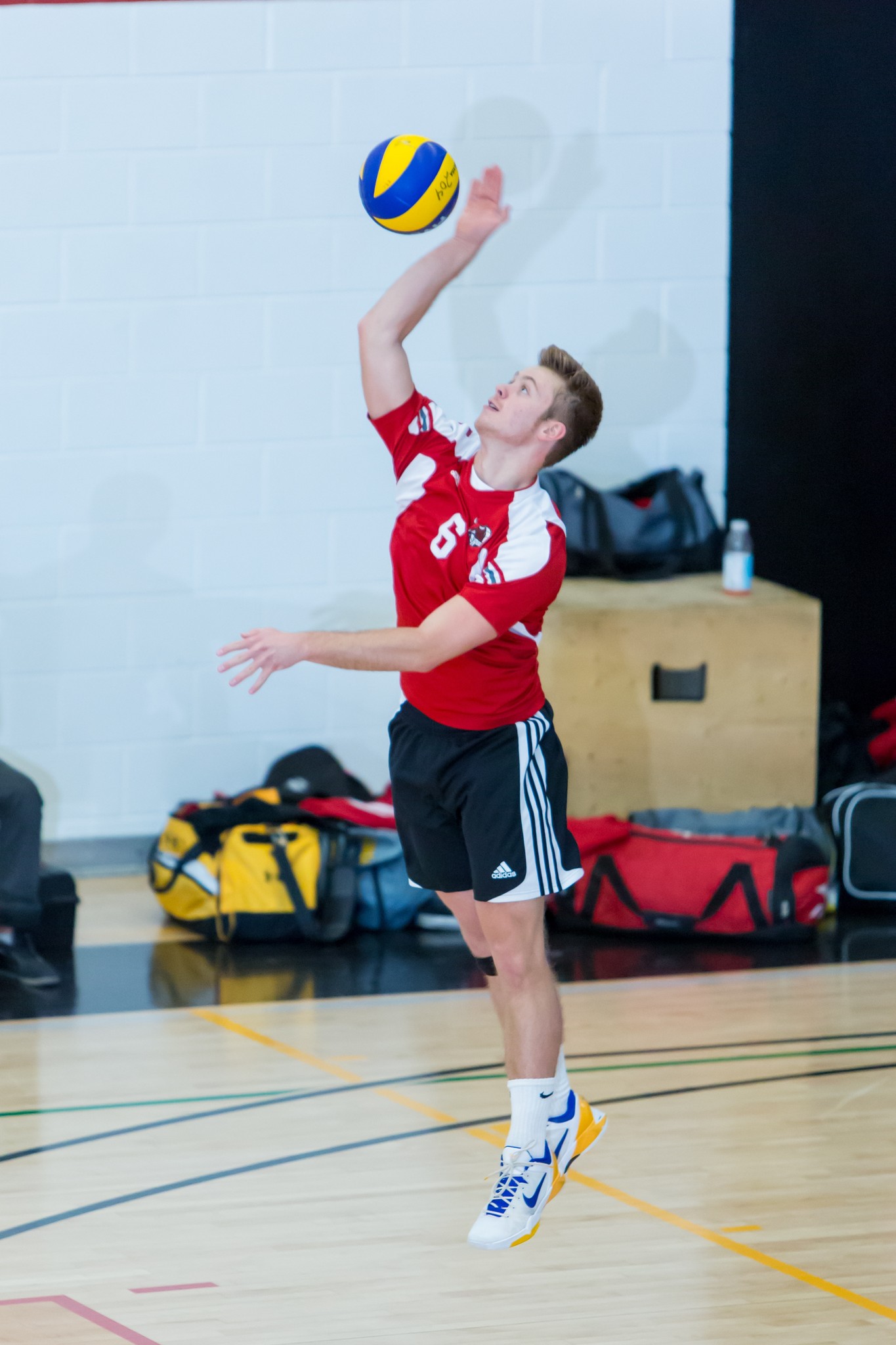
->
[822,780,896,912]
[145,782,435,953]
[544,815,828,938]
[539,463,730,580]
[627,805,837,884]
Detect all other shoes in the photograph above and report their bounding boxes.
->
[0,924,62,987]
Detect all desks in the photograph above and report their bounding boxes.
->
[536,568,822,820]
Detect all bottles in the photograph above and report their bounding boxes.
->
[722,518,752,595]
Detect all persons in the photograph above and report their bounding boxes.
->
[218,166,606,1246]
[0,759,59,986]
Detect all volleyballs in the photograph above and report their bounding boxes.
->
[358,134,460,234]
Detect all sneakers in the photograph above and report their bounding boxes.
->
[545,1088,608,1176]
[465,1136,565,1250]
